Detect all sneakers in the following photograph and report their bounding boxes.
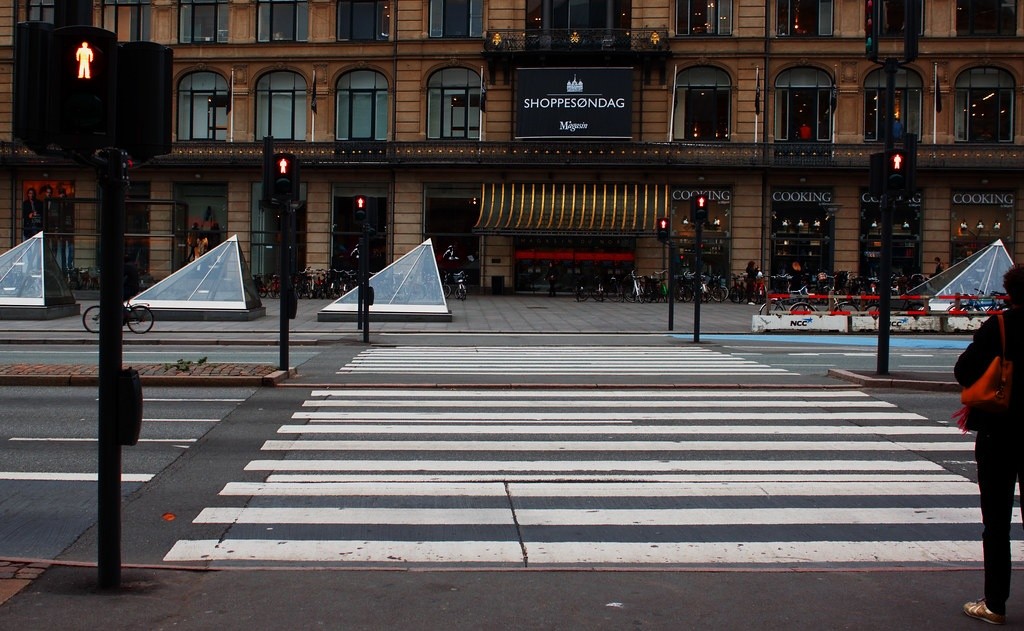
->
[963,598,1007,624]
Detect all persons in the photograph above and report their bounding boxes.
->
[745,261,759,306]
[934,257,945,278]
[953,264,1024,625]
[22,185,73,271]
[544,261,557,297]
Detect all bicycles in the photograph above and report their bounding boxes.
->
[83,295,154,334]
[62,267,100,289]
[755,272,929,302]
[252,273,281,298]
[674,273,745,305]
[573,272,667,304]
[289,266,359,300]
[442,271,468,301]
[759,285,1007,315]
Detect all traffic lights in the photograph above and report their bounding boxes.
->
[263,136,273,207]
[354,195,366,220]
[51,27,114,151]
[864,0,877,61]
[885,148,907,195]
[904,0,918,63]
[658,219,668,239]
[11,22,52,155]
[272,153,296,202]
[904,133,917,199]
[695,195,708,223]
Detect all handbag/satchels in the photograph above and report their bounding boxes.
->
[960,311,1013,412]
